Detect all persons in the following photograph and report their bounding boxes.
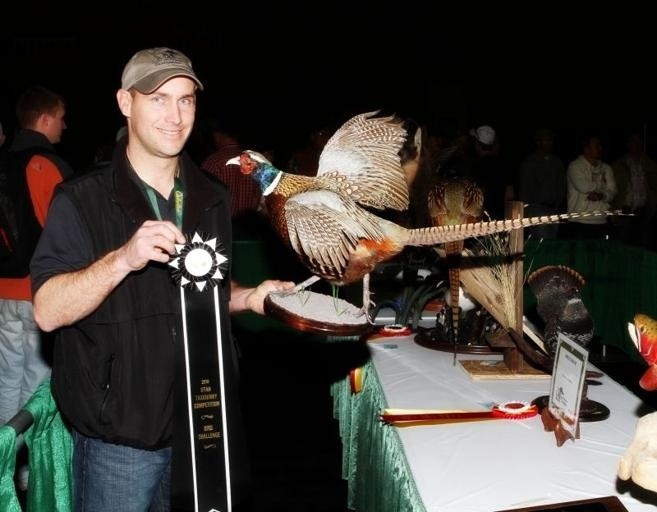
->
[191,110,655,252]
[31,46,294,512]
[0,88,76,424]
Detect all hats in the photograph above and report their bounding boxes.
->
[121,48,203,94]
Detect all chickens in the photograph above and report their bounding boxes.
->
[224,109,637,359]
